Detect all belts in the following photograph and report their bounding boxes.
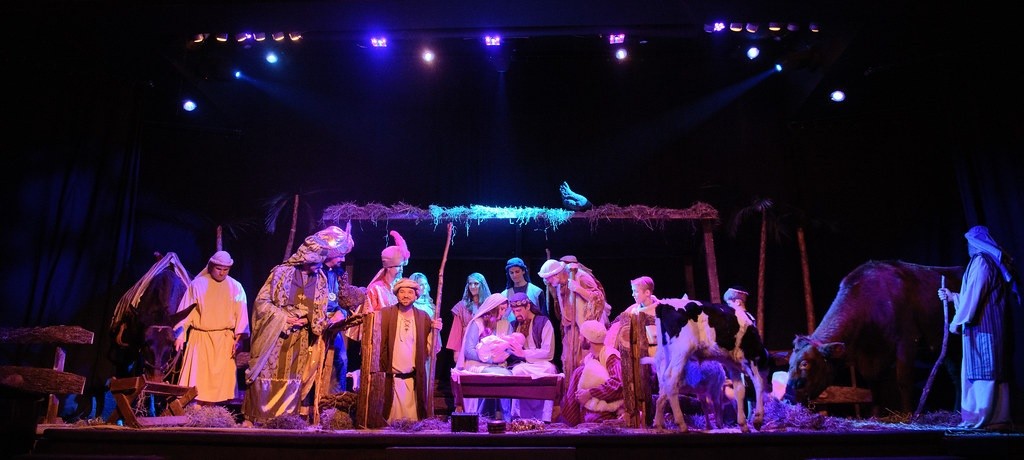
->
[392,371,416,379]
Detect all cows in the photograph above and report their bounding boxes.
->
[653,298,790,433]
[781,258,968,410]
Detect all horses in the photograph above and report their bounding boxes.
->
[63,294,197,424]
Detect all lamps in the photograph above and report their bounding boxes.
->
[730,23,820,32]
[192,32,302,43]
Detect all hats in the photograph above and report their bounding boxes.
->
[580,320,607,343]
[729,284,748,295]
[538,259,565,277]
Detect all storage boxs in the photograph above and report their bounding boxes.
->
[451,415,479,430]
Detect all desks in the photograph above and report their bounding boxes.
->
[449,373,565,413]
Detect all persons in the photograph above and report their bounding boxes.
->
[446,257,555,423]
[354,229,442,426]
[477,332,526,364]
[551,320,643,427]
[538,255,611,426]
[239,226,354,429]
[174,250,250,406]
[723,285,756,361]
[937,226,1023,431]
[608,275,659,358]
